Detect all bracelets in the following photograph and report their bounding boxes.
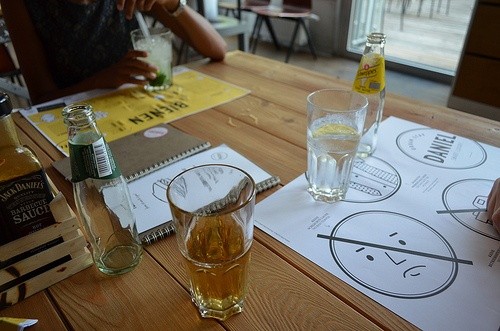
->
[163,0,186,18]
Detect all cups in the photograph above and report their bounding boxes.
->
[305,89,368,203]
[167,163,256,322]
[130,27,172,92]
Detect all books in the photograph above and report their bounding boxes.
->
[52,124,281,245]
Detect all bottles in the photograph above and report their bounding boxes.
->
[61,103,143,276]
[0,92,65,275]
[348,32,387,157]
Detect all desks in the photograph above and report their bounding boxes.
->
[0,49,500,331]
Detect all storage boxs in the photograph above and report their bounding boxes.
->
[0,173,95,311]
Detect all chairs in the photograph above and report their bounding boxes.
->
[160,0,318,68]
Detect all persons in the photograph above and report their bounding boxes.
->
[0,0,227,105]
[486,177,500,234]
